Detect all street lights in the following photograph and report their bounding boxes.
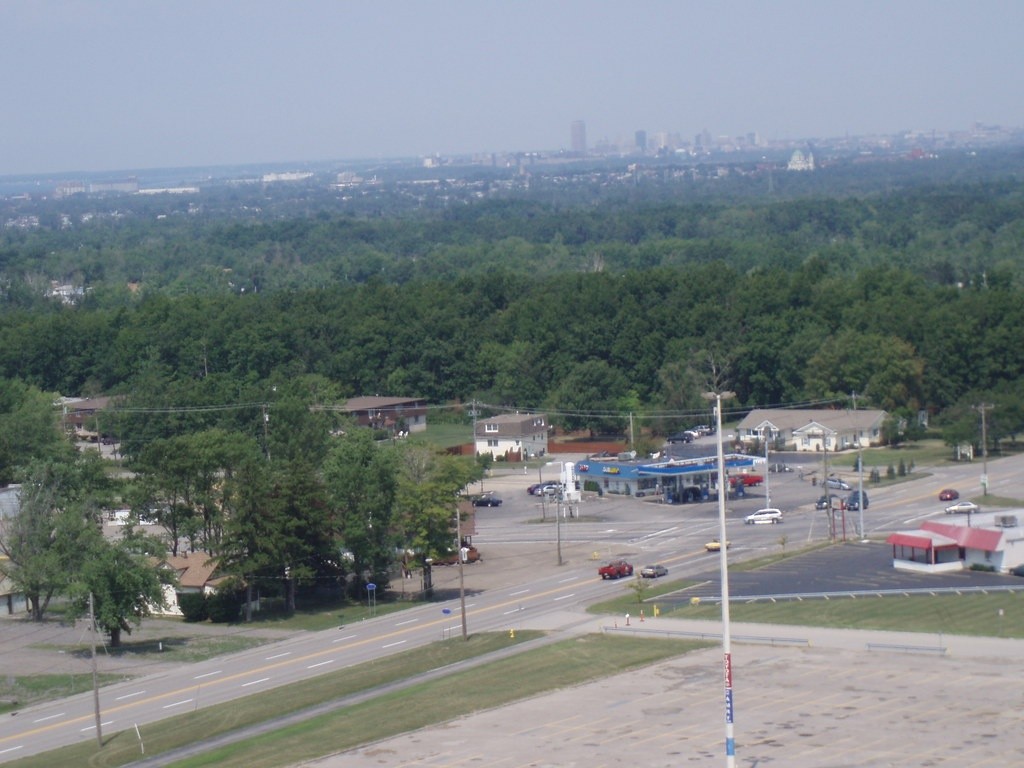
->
[700,391,736,768]
[447,502,469,641]
[546,461,566,517]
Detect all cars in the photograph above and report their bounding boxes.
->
[815,494,838,510]
[666,423,713,444]
[640,563,668,579]
[944,501,979,514]
[767,463,793,472]
[705,538,732,552]
[527,479,581,498]
[471,495,503,507]
[938,487,959,501]
[828,477,854,491]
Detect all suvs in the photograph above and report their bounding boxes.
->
[844,490,869,511]
[743,508,783,524]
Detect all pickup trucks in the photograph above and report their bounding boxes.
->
[598,559,633,579]
[729,472,764,487]
